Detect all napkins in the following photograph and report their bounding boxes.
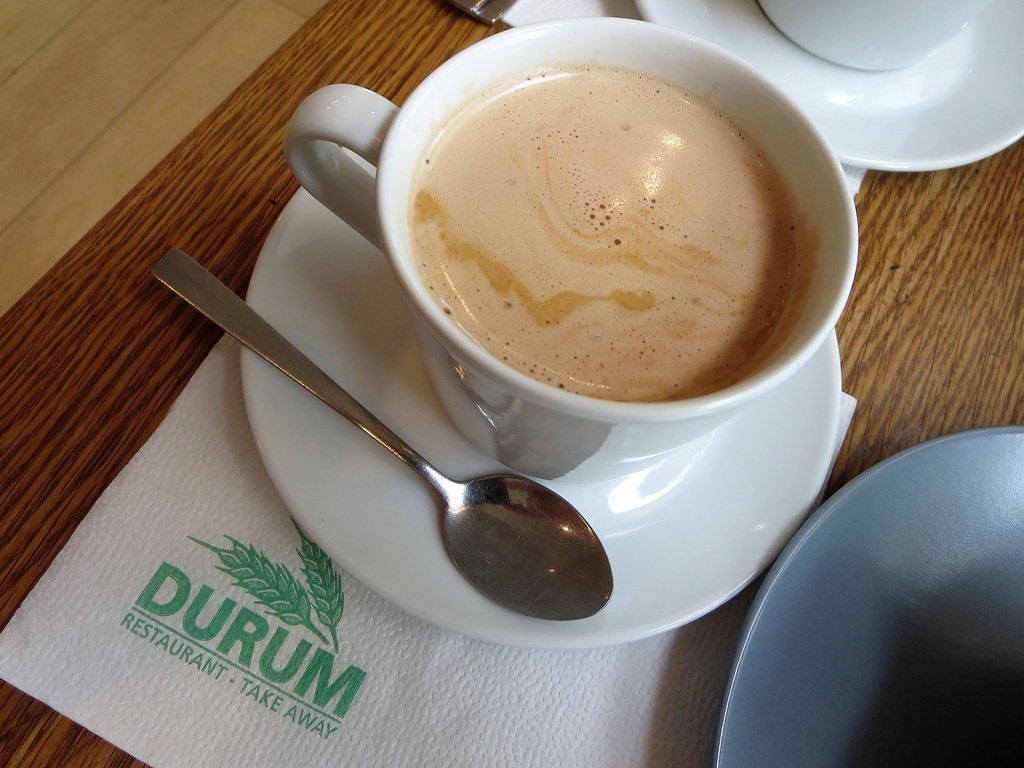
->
[0,317,858,768]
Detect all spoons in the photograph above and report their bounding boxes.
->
[148,245,613,622]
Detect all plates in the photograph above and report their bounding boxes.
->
[241,179,841,650]
[634,0,1024,172]
[711,425,1024,767]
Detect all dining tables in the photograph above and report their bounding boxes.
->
[2,1,1024,767]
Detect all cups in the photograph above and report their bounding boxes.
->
[757,0,988,72]
[285,14,860,481]
